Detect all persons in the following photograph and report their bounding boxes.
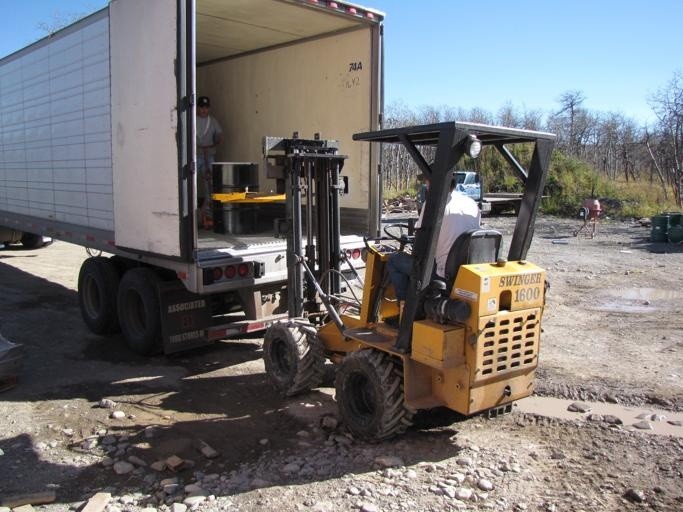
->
[383,162,482,327]
[196,95,225,231]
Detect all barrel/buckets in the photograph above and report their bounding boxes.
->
[211,162,260,235]
[661,212,683,242]
[651,216,669,242]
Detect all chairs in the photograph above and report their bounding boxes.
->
[430,229,502,296]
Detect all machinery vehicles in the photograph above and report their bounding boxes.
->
[256,119,559,447]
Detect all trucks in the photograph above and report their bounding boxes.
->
[0,0,389,364]
[411,170,552,220]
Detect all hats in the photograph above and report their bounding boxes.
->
[415,163,434,181]
[197,95,211,107]
